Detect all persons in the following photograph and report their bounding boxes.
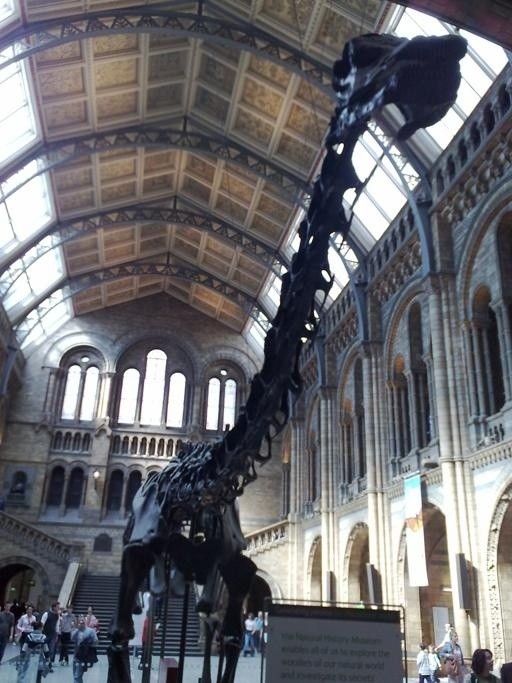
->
[241,607,269,657]
[415,623,512,682]
[127,587,163,671]
[0,598,101,683]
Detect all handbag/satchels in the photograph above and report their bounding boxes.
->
[440,657,461,676]
